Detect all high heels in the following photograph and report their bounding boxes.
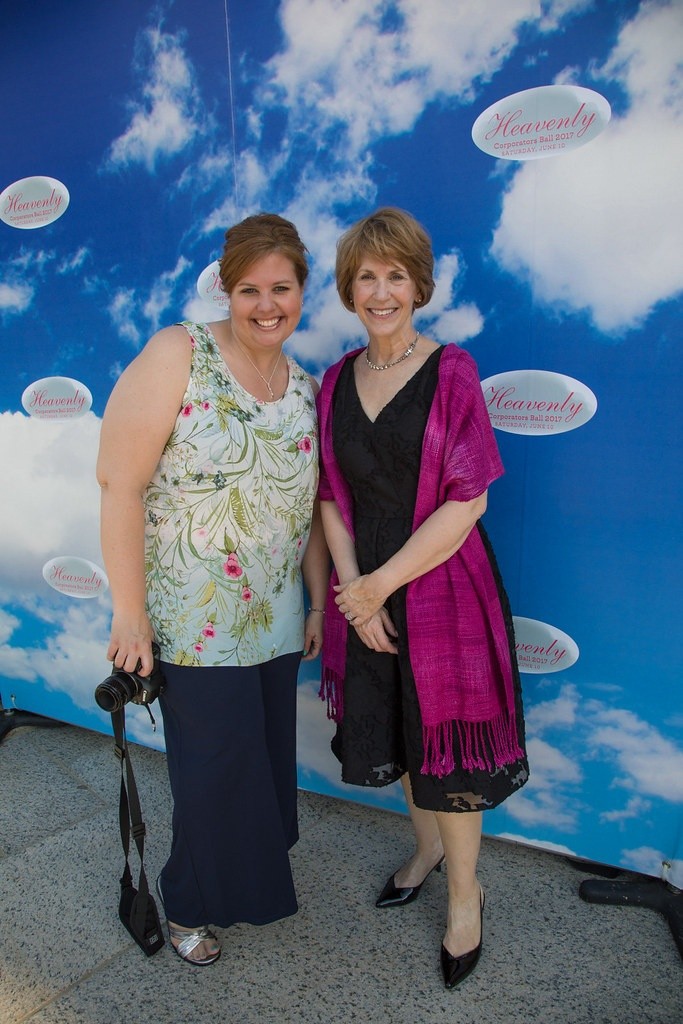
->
[440,883,486,989]
[375,850,446,908]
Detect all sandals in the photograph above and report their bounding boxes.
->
[156,872,221,967]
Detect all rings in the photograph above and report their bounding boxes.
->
[346,612,353,620]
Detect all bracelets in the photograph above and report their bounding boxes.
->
[308,608,326,615]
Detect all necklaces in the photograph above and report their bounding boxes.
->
[365,330,419,370]
[230,324,283,399]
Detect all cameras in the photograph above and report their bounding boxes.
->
[95,641,167,712]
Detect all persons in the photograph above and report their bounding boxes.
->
[97,214,332,966]
[314,207,529,989]
